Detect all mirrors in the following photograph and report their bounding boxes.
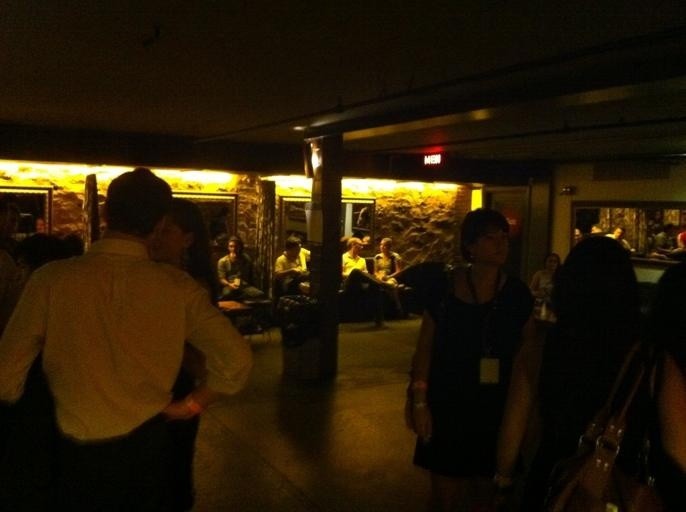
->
[172,196,378,297]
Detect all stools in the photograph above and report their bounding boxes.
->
[218,300,269,348]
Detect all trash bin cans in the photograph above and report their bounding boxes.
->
[280,295,328,386]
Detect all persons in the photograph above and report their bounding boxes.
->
[341,236,399,330]
[373,237,413,317]
[148,197,218,512]
[573,207,686,262]
[216,235,265,334]
[530,252,562,351]
[0,167,255,512]
[274,234,311,302]
[614,257,686,510]
[486,235,646,512]
[0,190,85,512]
[404,206,539,511]
[207,205,230,300]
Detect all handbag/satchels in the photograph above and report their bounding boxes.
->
[552,336,659,512]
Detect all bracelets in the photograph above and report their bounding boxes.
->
[411,398,430,410]
[183,390,205,416]
[488,472,517,490]
[411,377,429,392]
